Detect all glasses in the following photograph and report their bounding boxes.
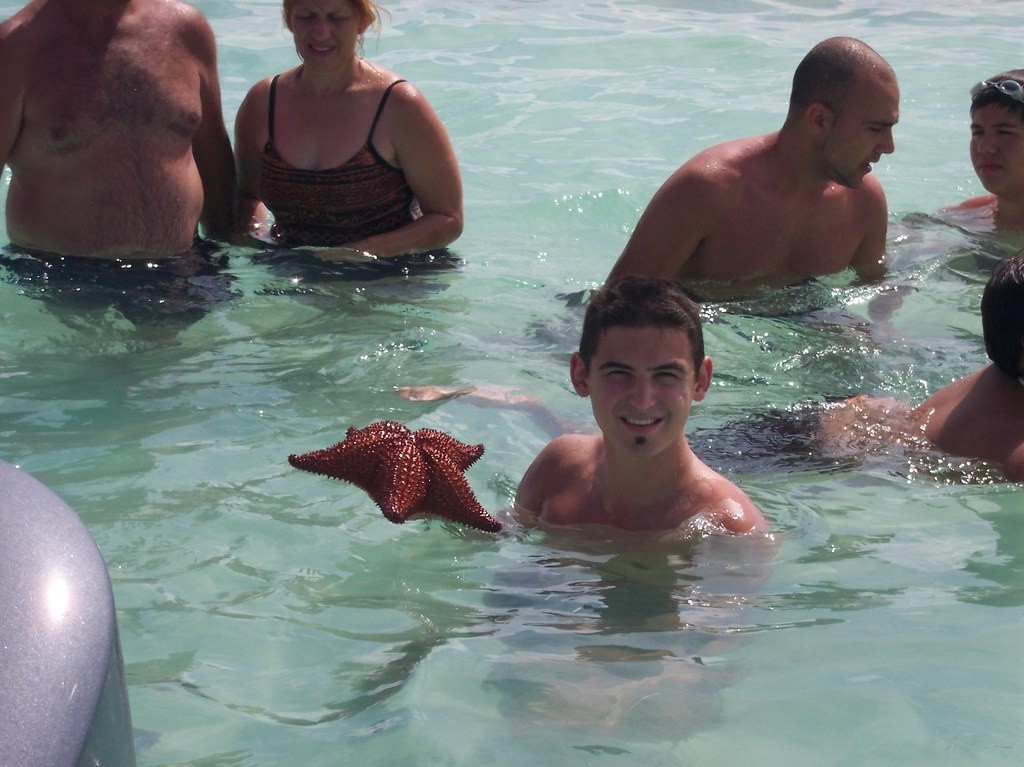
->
[969,80,1024,102]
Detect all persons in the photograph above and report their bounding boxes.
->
[516,277,764,534]
[912,254,1024,481]
[0,1,235,255]
[944,71,1024,224]
[604,38,900,284]
[232,2,464,258]
